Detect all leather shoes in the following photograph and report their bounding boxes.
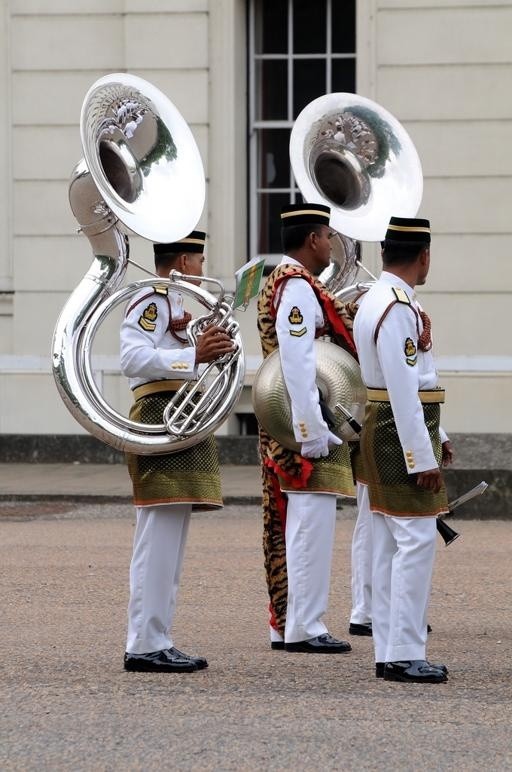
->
[271,632,352,653]
[349,623,432,637]
[124,646,209,672]
[376,660,449,682]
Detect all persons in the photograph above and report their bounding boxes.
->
[354,216,456,684]
[118,230,236,674]
[258,205,360,653]
[349,242,432,637]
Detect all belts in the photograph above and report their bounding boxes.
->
[366,386,445,404]
[132,380,205,403]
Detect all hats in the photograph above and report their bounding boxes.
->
[380,217,430,249]
[153,230,206,253]
[278,203,330,227]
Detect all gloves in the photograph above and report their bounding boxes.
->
[300,431,343,459]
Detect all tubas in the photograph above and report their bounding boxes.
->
[290,89,424,319]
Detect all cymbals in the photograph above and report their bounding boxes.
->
[250,339,370,453]
